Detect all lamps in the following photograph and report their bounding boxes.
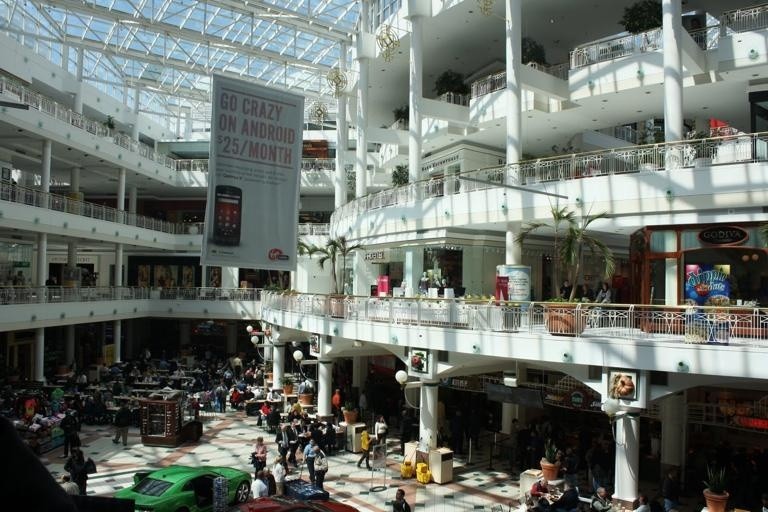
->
[602,400,624,446]
[395,370,419,408]
[246,326,317,381]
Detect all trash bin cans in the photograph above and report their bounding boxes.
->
[501,306,521,329]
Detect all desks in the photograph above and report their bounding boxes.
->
[0,357,343,456]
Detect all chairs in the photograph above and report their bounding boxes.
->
[491,491,531,512]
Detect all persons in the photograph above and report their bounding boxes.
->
[182,271,192,288]
[434,278,448,295]
[354,425,372,471]
[357,389,369,420]
[0,269,33,306]
[208,268,220,288]
[390,489,412,512]
[397,409,412,456]
[46,273,62,303]
[576,283,594,301]
[331,387,341,427]
[419,271,430,293]
[590,282,611,326]
[507,418,768,511]
[373,413,389,447]
[558,280,573,302]
[161,273,171,288]
[81,273,93,288]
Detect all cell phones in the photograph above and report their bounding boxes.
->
[213,185,242,246]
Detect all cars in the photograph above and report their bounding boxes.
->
[111,465,252,512]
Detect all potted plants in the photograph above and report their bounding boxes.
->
[515,193,618,336]
[310,237,364,317]
[540,439,560,481]
[703,464,729,512]
[341,401,359,424]
